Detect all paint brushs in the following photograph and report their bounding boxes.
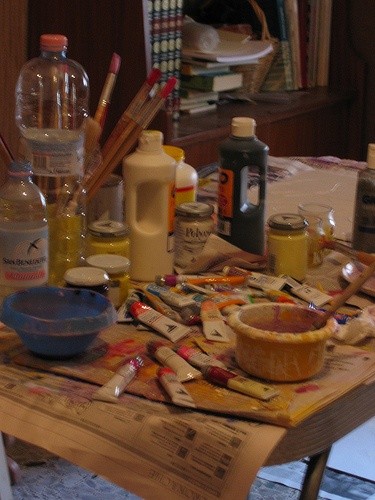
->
[329,238,375,265]
[69,52,176,204]
[308,266,375,328]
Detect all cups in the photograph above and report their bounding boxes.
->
[88,175,124,224]
[47,204,87,288]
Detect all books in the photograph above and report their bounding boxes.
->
[148,0,331,129]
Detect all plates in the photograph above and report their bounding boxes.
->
[341,263,375,296]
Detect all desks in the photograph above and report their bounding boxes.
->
[0,159,375,500]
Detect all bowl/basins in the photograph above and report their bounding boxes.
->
[226,302,339,382]
[1,286,116,358]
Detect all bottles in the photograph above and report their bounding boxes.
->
[216,116,270,256]
[16,36,89,289]
[298,202,336,267]
[121,129,177,283]
[0,160,48,331]
[353,144,375,254]
[161,145,198,206]
